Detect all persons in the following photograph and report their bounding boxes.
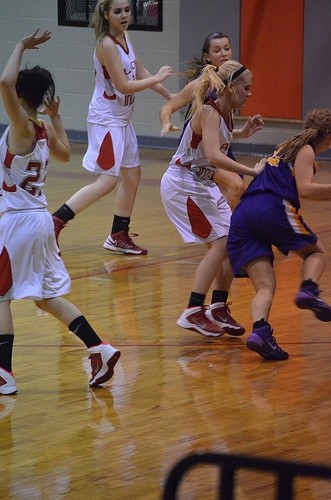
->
[226,109,331,360]
[161,33,265,209]
[50,0,178,257]
[0,30,120,395]
[160,60,267,336]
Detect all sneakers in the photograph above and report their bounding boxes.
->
[51,215,66,257]
[0,366,18,394]
[295,288,331,322]
[175,305,225,337]
[246,318,290,361]
[102,227,147,255]
[86,340,121,387]
[203,302,247,336]
